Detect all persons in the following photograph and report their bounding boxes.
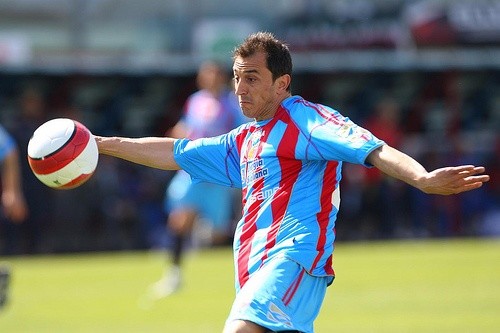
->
[92,31,498,333]
[138,62,253,301]
[0,112,28,307]
[353,93,417,245]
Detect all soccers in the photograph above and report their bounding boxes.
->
[28,117,100,191]
